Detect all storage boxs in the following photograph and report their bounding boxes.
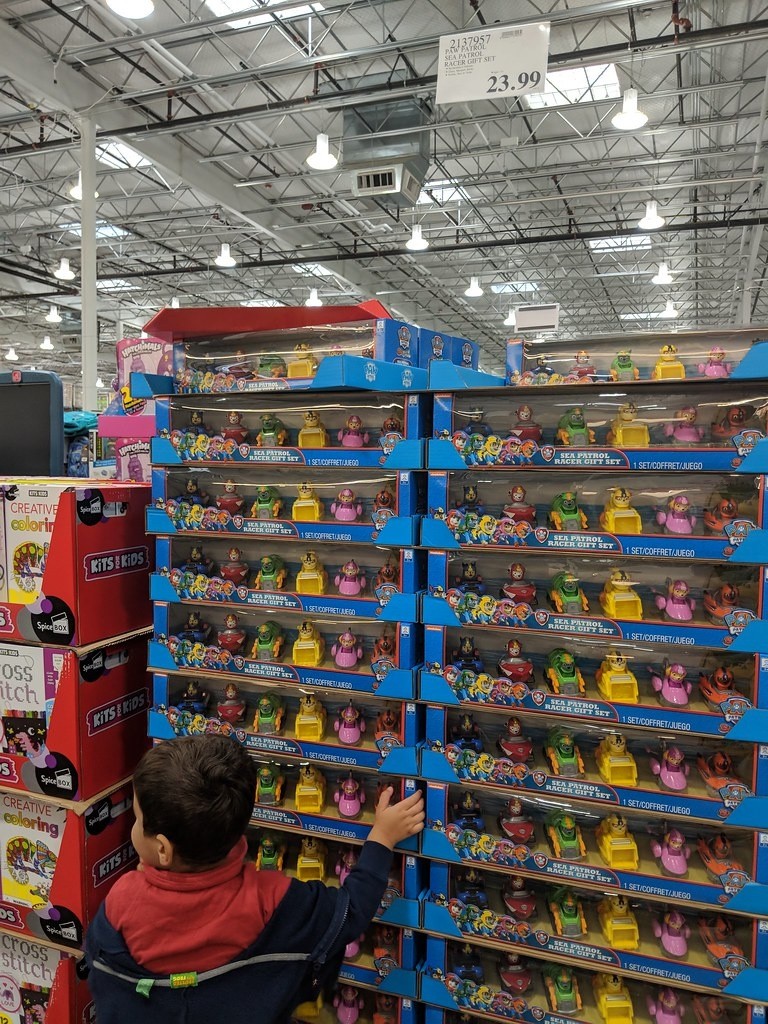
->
[0,316,768,1024]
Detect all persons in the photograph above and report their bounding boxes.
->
[84,733,425,1024]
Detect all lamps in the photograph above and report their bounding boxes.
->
[96,378,105,388]
[213,243,237,268]
[610,86,648,131]
[70,170,101,201]
[405,223,429,251]
[54,257,76,281]
[306,133,338,171]
[503,308,516,325]
[304,287,323,307]
[4,347,19,361]
[638,200,665,230]
[44,306,62,323]
[465,276,483,297]
[651,262,673,284]
[40,335,54,350]
[660,300,678,318]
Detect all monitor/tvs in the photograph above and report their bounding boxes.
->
[0,371,65,477]
